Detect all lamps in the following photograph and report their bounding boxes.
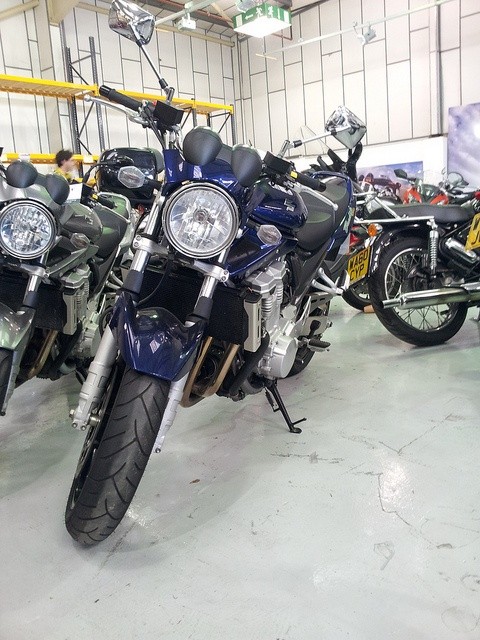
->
[232,4,291,39]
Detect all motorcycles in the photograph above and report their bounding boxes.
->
[338,168,480,314]
[0,146,164,418]
[347,192,479,346]
[0,0,357,547]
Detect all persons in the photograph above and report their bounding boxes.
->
[52,149,103,201]
